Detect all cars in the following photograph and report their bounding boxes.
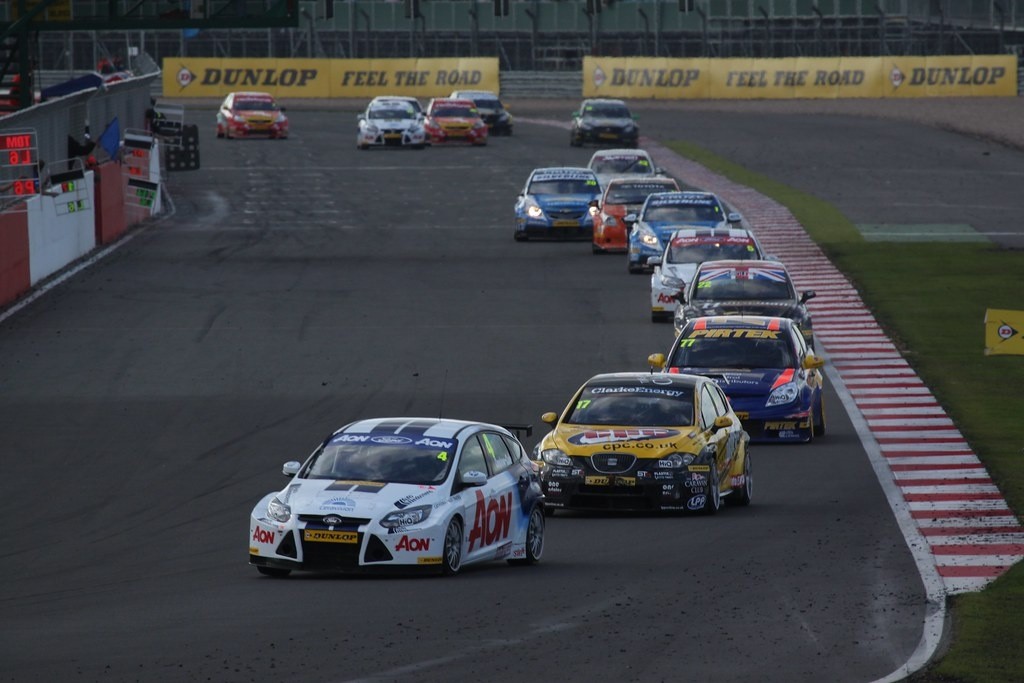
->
[587,148,668,198]
[532,370,753,515]
[586,177,681,254]
[450,91,515,138]
[623,191,742,274]
[646,315,827,442]
[569,98,641,150]
[216,91,289,140]
[355,100,426,149]
[667,259,818,351]
[512,167,604,241]
[420,98,488,146]
[646,227,779,323]
[373,95,426,122]
[247,416,547,579]
